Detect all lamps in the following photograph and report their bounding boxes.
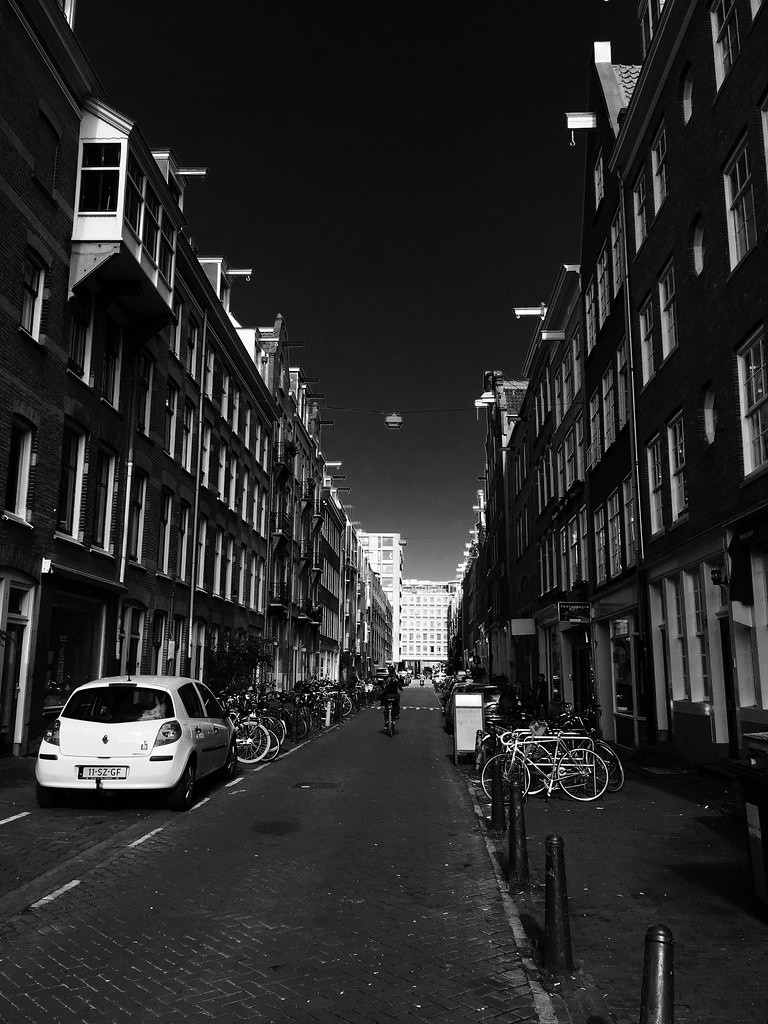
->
[269,636,279,647]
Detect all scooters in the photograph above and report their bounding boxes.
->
[418,678,426,687]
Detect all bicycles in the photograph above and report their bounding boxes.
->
[213,675,379,765]
[476,703,629,803]
[384,696,397,738]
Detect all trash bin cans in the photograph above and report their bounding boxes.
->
[730,731,768,904]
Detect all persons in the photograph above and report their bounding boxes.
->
[420,674,425,685]
[381,666,404,730]
[536,673,549,718]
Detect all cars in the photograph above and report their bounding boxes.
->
[32,673,240,811]
[463,683,522,728]
[431,672,493,730]
[397,670,412,686]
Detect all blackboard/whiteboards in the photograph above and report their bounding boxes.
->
[454,692,485,753]
